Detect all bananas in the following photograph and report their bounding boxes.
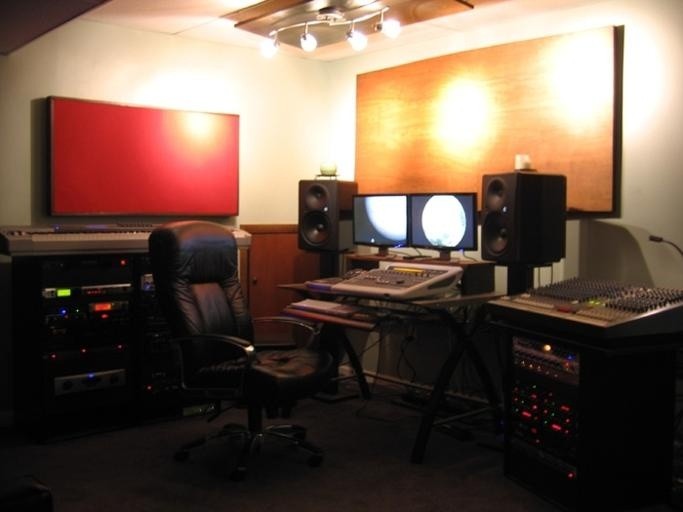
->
[290,298,363,317]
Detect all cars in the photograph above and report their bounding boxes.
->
[320,160,336,175]
[514,154,533,171]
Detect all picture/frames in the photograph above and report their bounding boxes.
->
[280,254,508,462]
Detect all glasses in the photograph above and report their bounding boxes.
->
[261,0,405,60]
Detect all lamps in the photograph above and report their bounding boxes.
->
[148,221,335,477]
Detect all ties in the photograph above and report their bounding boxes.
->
[352,193,408,262]
[409,192,478,266]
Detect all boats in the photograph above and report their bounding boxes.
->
[481,174,566,265]
[297,179,359,254]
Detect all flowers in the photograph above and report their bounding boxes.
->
[648,235,681,257]
